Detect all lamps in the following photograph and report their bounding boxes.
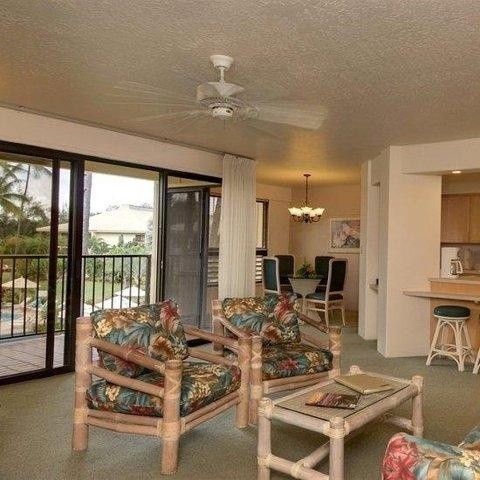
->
[287,168,325,223]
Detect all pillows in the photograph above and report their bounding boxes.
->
[89,298,190,385]
[223,297,302,351]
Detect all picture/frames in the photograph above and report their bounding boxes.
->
[327,217,361,255]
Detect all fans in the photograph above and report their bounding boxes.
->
[109,54,328,134]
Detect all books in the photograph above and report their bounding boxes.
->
[333,373,393,394]
[304,392,363,410]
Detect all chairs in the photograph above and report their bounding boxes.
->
[211,295,339,428]
[72,296,251,474]
[261,253,349,329]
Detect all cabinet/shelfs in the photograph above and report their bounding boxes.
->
[441,192,480,245]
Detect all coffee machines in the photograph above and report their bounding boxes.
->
[441,247,463,279]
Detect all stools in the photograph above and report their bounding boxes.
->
[424,304,475,372]
